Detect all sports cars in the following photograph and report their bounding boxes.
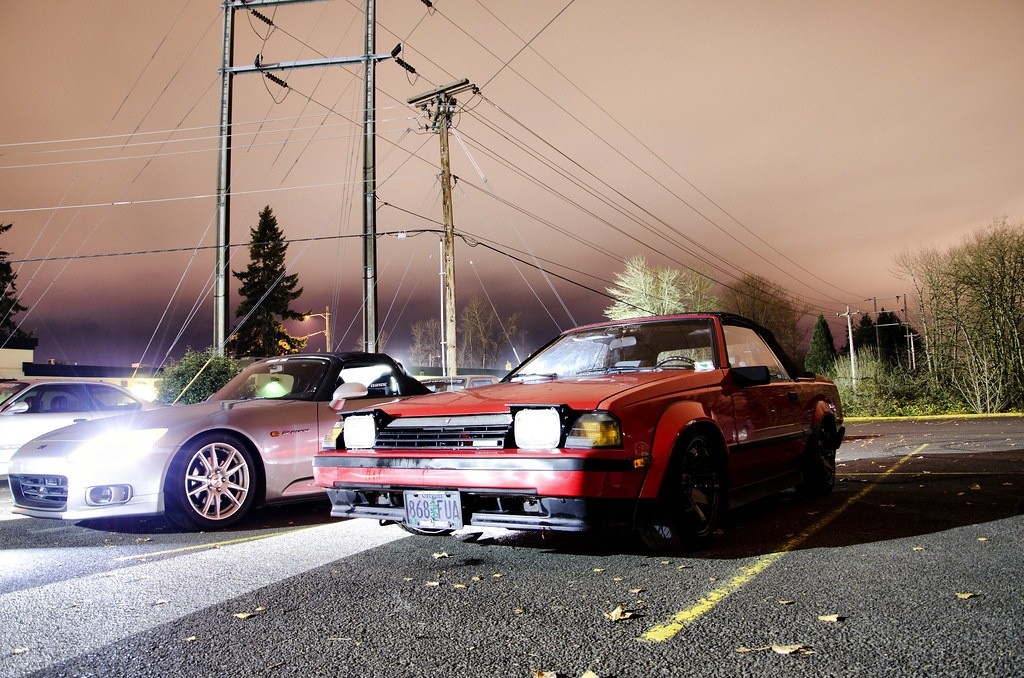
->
[7,353,433,531]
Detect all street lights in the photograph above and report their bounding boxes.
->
[297,304,331,353]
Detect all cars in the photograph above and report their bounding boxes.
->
[417,375,502,396]
[0,375,190,484]
[313,307,846,551]
